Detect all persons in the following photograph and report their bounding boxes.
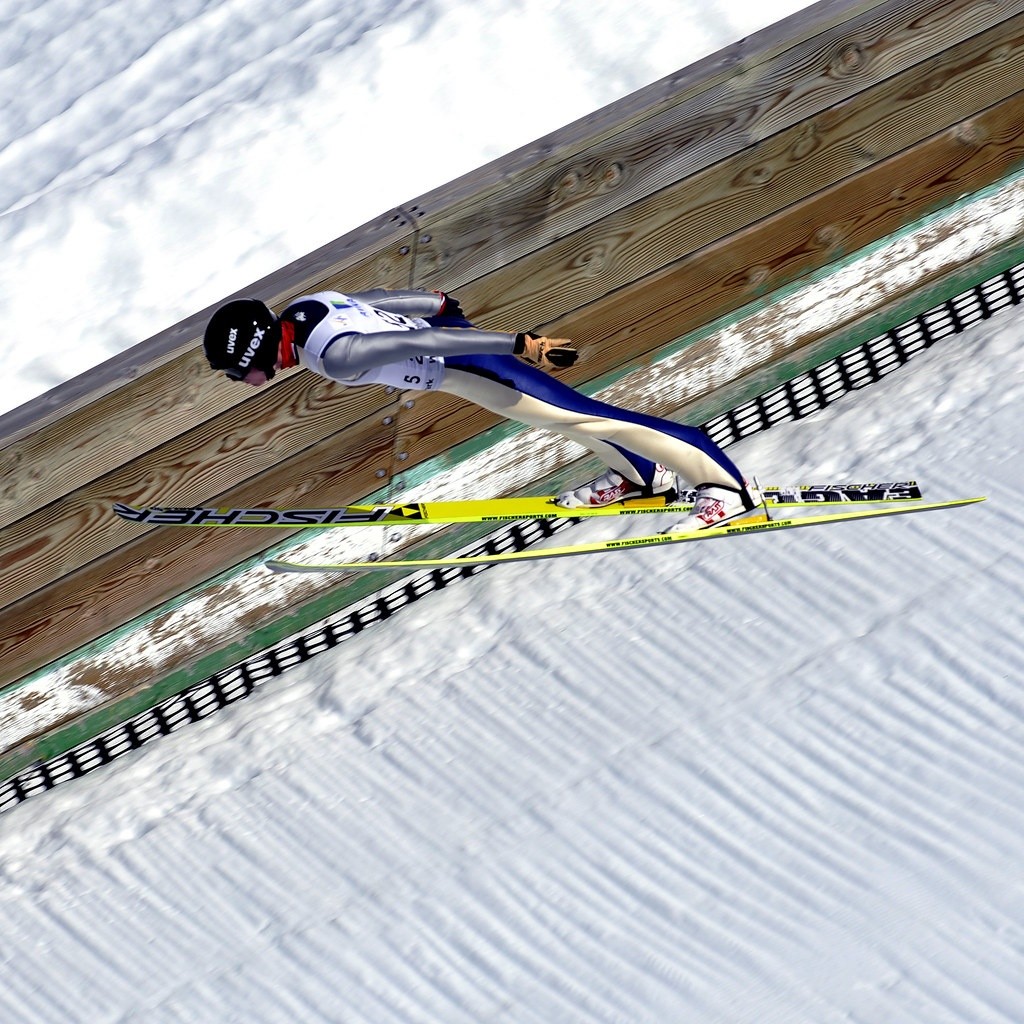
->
[205,289,769,535]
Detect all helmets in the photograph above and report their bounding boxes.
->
[202,298,280,381]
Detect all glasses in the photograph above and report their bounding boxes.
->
[220,366,246,383]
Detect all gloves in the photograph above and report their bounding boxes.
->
[438,291,466,318]
[513,330,578,371]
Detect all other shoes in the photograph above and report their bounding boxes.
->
[662,477,764,535]
[552,464,676,509]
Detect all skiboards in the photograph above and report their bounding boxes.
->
[104,477,987,576]
